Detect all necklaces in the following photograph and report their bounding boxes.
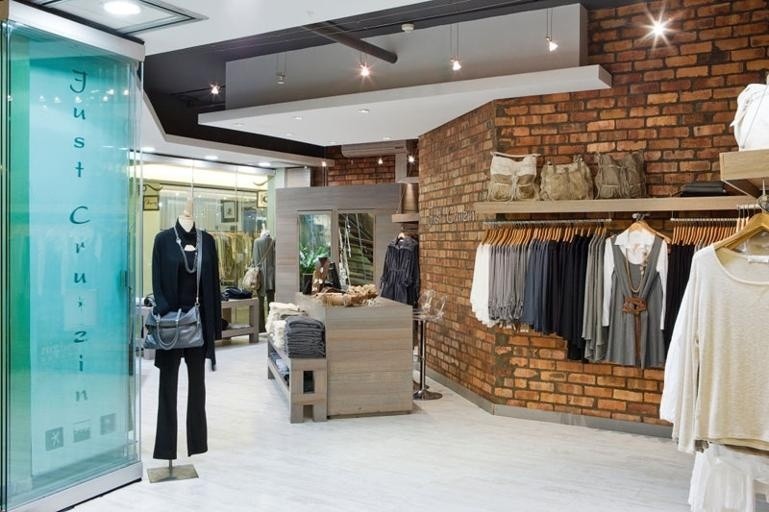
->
[173,224,198,274]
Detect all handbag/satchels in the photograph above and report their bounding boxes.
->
[539,154,593,199]
[731,84,768,150]
[242,270,261,290]
[488,151,539,201]
[143,306,204,350]
[595,151,647,198]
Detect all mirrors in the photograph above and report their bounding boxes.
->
[297,209,331,292]
[337,210,373,292]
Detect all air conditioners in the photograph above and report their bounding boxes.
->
[341,139,414,158]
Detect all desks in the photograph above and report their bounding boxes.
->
[135,297,259,360]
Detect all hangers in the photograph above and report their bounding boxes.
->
[481,194,769,250]
[396,226,416,240]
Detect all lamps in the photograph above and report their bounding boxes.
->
[211,1,687,94]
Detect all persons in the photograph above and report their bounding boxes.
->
[153,207,223,460]
[253,229,274,333]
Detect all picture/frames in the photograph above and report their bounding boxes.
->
[221,199,238,222]
[142,194,160,210]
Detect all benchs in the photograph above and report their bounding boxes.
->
[265,332,328,423]
